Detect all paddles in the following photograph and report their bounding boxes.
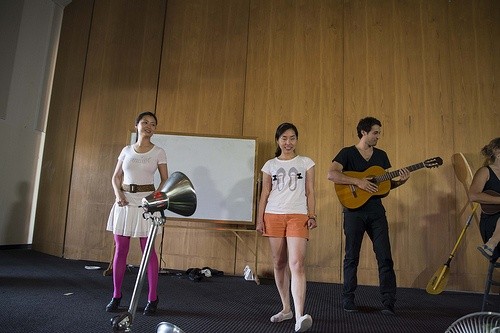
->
[425,202,481,295]
[451,152,480,226]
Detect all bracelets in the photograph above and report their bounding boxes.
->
[309,215,317,222]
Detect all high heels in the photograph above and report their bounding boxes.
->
[105,291,123,312]
[143,296,159,316]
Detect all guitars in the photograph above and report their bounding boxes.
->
[334,156,443,209]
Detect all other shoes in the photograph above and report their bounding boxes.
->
[343,304,360,313]
[270,310,293,322]
[477,244,494,261]
[382,304,395,316]
[295,313,313,333]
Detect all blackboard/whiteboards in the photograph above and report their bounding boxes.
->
[126,128,259,225]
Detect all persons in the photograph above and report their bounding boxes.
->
[327,117,410,315]
[106,112,168,316]
[468,138,500,261]
[257,123,318,333]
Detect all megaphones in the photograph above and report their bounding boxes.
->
[138,171,198,219]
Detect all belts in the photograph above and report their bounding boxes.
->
[121,184,156,193]
